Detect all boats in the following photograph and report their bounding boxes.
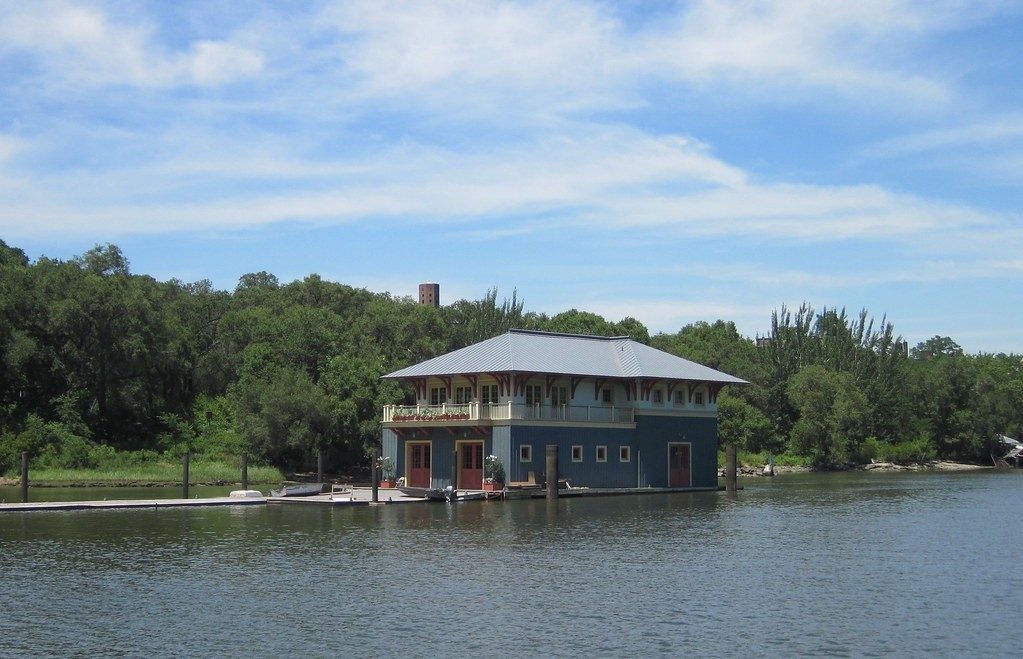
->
[269,483,327,497]
[395,486,458,498]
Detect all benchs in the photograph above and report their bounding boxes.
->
[330,485,354,501]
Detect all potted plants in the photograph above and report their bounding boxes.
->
[482,460,506,490]
[376,456,396,488]
[391,408,470,420]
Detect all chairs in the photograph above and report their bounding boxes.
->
[395,476,406,488]
